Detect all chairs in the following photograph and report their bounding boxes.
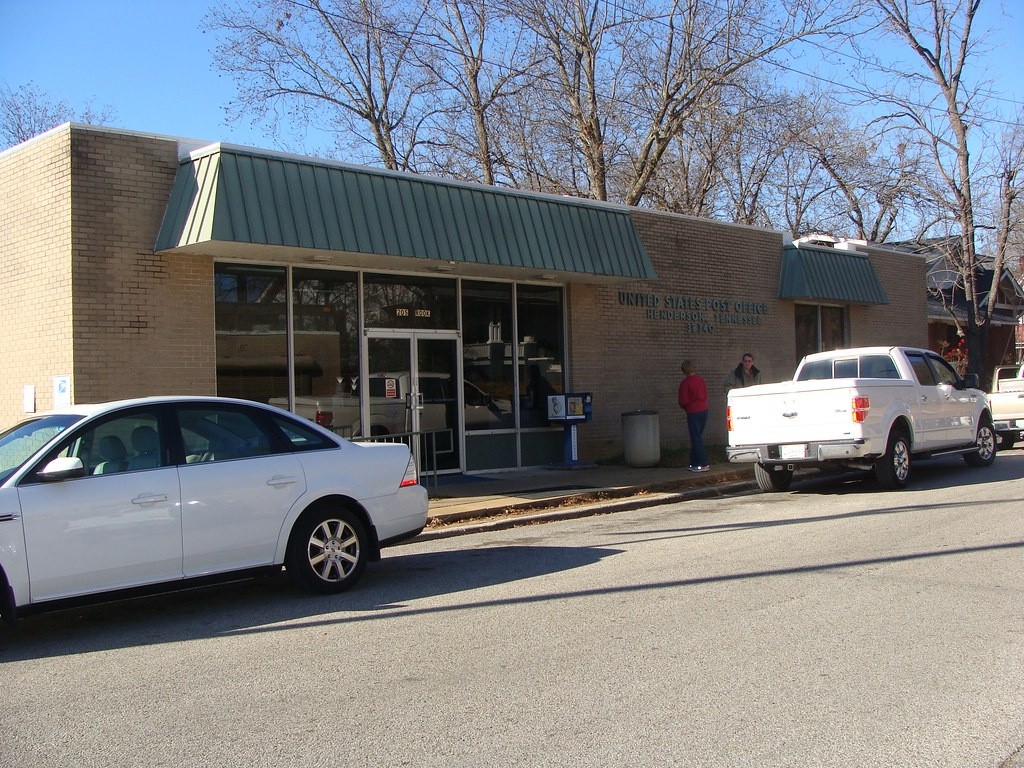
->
[126,426,161,470]
[870,360,893,378]
[810,365,831,379]
[93,434,125,474]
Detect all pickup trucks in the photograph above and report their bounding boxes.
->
[987,364,1024,450]
[268,370,512,442]
[725,346,998,493]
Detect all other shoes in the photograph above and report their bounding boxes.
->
[691,466,710,471]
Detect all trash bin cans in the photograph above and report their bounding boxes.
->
[622,410,660,467]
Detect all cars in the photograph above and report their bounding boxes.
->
[0,395,428,638]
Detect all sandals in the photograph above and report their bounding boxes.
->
[689,465,694,470]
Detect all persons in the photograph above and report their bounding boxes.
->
[678,360,710,473]
[724,353,767,394]
[525,367,556,419]
